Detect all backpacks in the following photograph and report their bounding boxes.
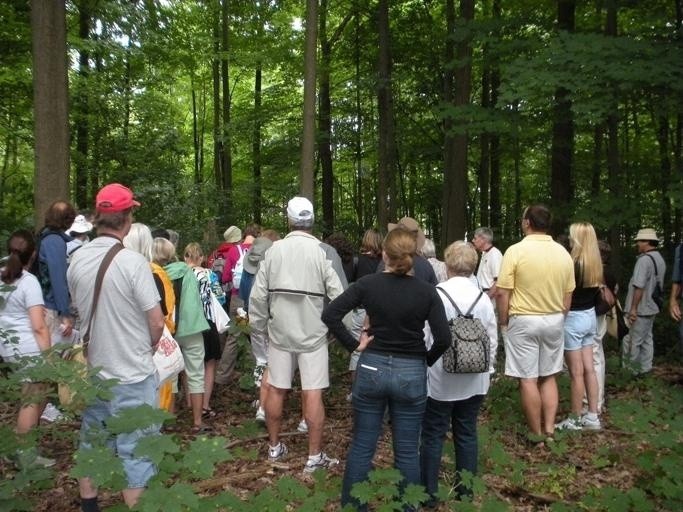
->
[434,286,489,373]
[230,245,247,289]
[210,249,225,273]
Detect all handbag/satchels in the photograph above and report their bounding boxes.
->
[650,281,664,313]
[57,344,88,405]
[594,287,615,316]
[604,298,628,341]
[210,293,230,335]
[152,324,184,384]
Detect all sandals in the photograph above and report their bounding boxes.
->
[201,408,213,415]
[191,424,212,432]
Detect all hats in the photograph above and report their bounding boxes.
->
[64,214,93,237]
[387,217,425,249]
[243,237,272,274]
[95,183,140,212]
[223,226,241,243]
[286,196,313,221]
[633,228,660,242]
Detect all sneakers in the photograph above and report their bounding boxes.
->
[563,418,600,431]
[267,441,288,462]
[302,453,339,474]
[254,406,264,428]
[40,401,67,424]
[296,419,307,432]
[554,417,581,430]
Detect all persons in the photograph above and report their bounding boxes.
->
[1,182,681,512]
